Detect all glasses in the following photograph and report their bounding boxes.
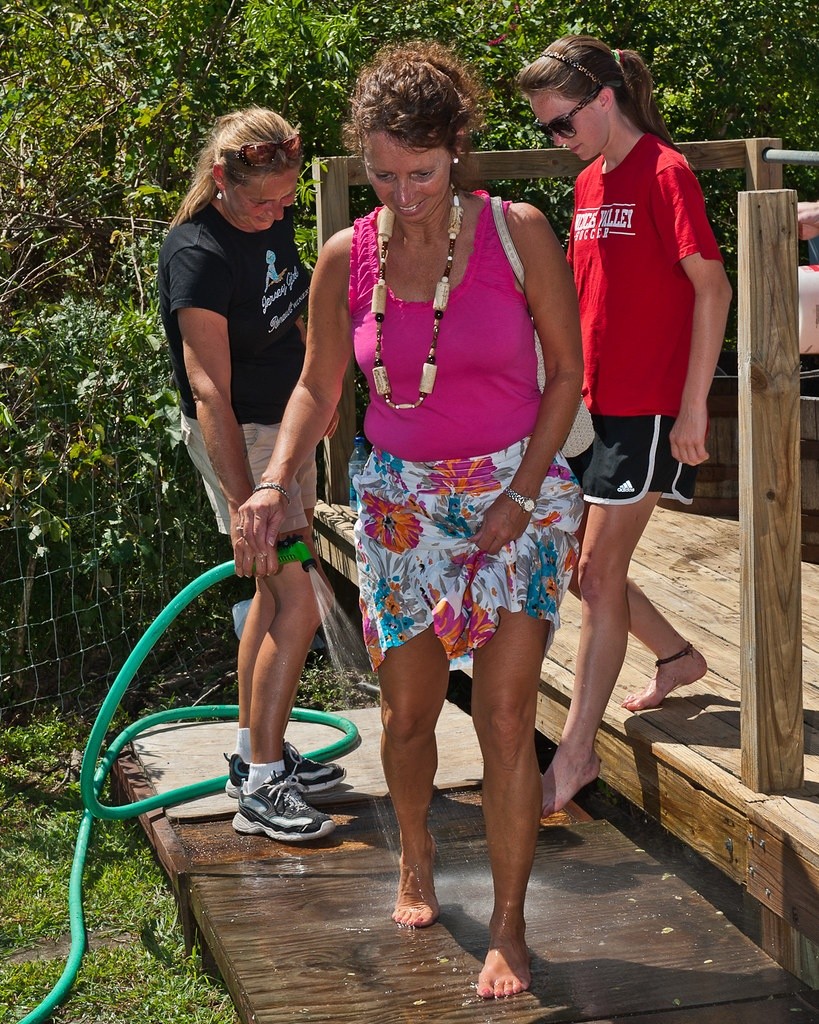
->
[533,85,603,140]
[220,133,301,168]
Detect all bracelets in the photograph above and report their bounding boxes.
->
[252,483,291,504]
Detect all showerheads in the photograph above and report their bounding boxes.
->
[250,540,316,573]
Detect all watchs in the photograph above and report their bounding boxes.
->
[503,486,537,514]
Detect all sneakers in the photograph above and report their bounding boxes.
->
[232,764,335,841]
[224,739,346,799]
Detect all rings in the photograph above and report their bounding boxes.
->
[235,527,243,531]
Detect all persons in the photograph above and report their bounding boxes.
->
[797,201,819,239]
[158,108,348,842]
[236,40,586,998]
[515,35,731,819]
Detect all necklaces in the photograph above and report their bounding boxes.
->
[371,180,464,409]
